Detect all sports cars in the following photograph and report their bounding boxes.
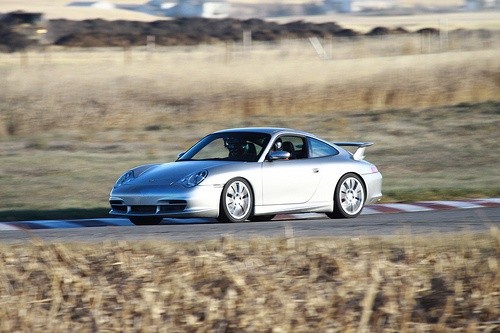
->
[107,126,382,225]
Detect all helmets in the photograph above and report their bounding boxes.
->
[263,135,282,153]
[224,134,249,152]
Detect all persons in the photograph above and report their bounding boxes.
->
[263,135,290,159]
[227,136,249,157]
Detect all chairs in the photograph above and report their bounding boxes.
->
[283,141,297,159]
[242,143,258,162]
